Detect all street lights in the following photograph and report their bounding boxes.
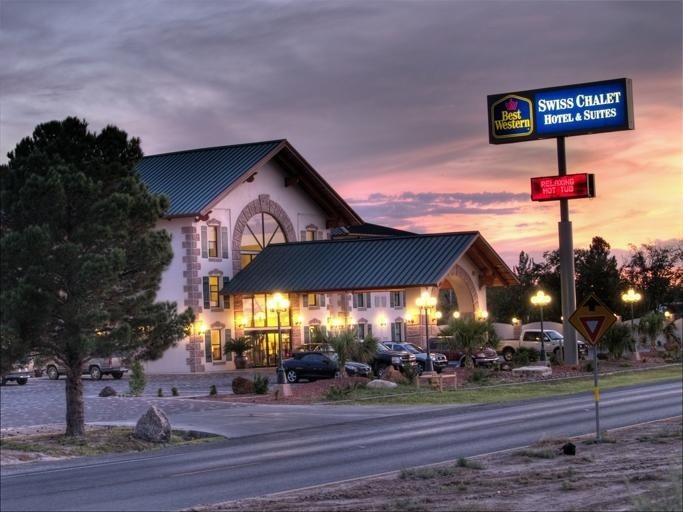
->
[416,292,437,371]
[268,293,289,384]
[530,291,551,362]
[622,290,641,353]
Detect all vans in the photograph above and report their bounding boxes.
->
[424,336,499,368]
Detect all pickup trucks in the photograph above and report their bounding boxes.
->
[497,329,588,361]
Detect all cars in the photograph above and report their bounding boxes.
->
[0,357,33,386]
[45,350,128,380]
[283,342,448,384]
